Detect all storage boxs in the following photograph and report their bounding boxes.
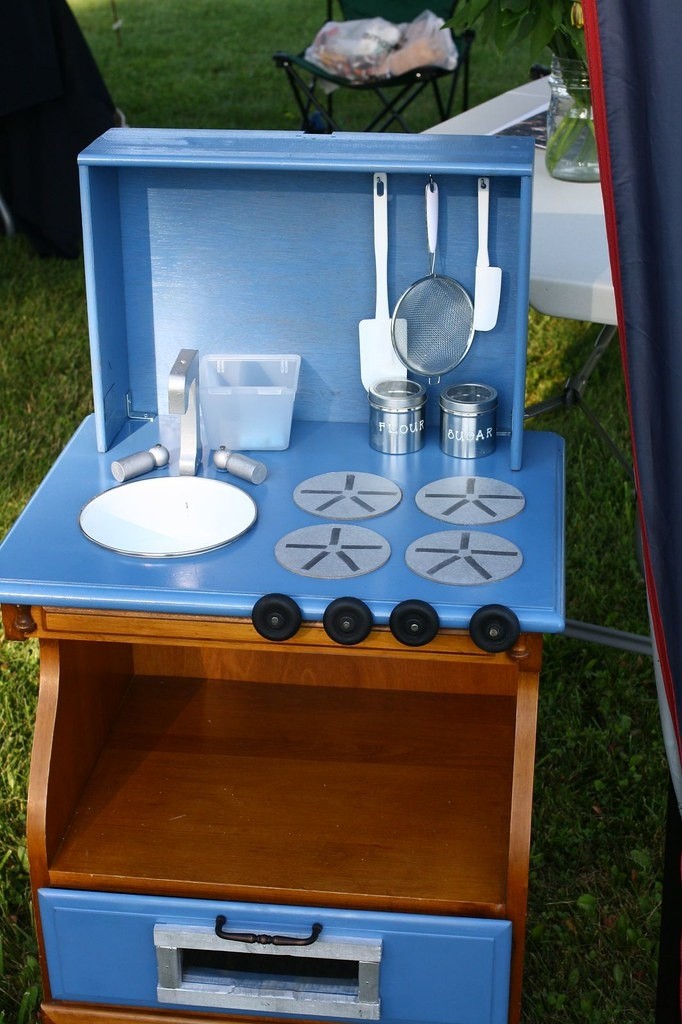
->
[196,353,302,451]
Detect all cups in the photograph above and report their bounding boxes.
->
[367,378,428,455]
[439,383,499,459]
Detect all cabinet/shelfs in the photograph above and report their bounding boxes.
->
[0,127,567,1023]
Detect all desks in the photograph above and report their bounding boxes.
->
[419,75,655,663]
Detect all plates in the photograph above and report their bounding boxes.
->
[79,476,257,557]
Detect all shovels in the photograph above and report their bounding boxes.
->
[356,172,408,394]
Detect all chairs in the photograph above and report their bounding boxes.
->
[273,0,476,133]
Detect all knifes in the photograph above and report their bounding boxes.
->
[473,178,501,331]
